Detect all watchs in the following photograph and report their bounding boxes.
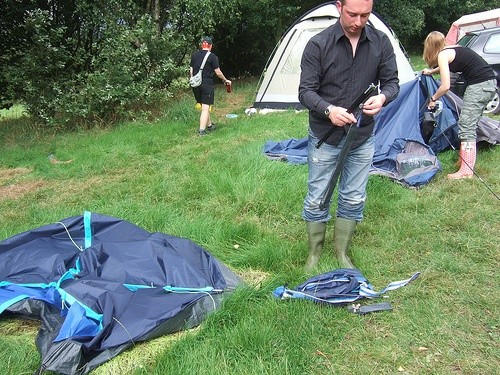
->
[325,105,335,115]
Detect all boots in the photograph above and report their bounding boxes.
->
[447,140,477,180]
[303,222,326,270]
[334,217,356,270]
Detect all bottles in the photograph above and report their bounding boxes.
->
[226,83,231,93]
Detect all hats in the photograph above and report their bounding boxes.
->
[201,36,213,45]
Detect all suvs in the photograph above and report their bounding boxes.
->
[434,26,500,116]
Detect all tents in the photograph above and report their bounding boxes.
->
[251,1,418,110]
[444,8,500,45]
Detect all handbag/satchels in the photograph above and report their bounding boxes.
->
[189,71,202,88]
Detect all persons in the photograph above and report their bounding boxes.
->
[298,0,400,270]
[190,38,231,134]
[423,31,497,178]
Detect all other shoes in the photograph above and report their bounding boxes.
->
[199,123,215,136]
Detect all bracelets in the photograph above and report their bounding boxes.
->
[431,97,436,102]
[223,78,226,82]
[428,71,430,74]
